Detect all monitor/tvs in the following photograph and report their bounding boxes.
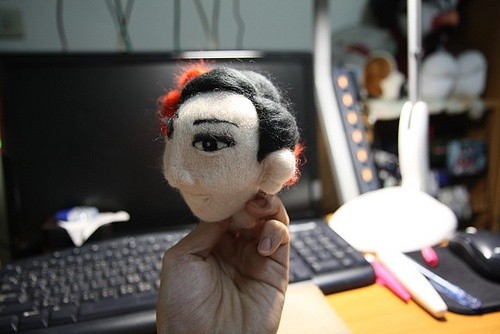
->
[1,49,323,260]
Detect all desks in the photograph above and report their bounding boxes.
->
[325,212,500,334]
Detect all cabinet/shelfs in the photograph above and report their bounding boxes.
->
[310,0,500,230]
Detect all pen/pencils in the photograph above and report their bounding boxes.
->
[363,253,411,303]
[407,253,482,309]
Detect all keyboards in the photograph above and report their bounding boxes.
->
[0,219,374,334]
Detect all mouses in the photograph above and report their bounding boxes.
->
[449,227,500,281]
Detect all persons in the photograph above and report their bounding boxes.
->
[155,191,290,334]
[157,59,304,222]
